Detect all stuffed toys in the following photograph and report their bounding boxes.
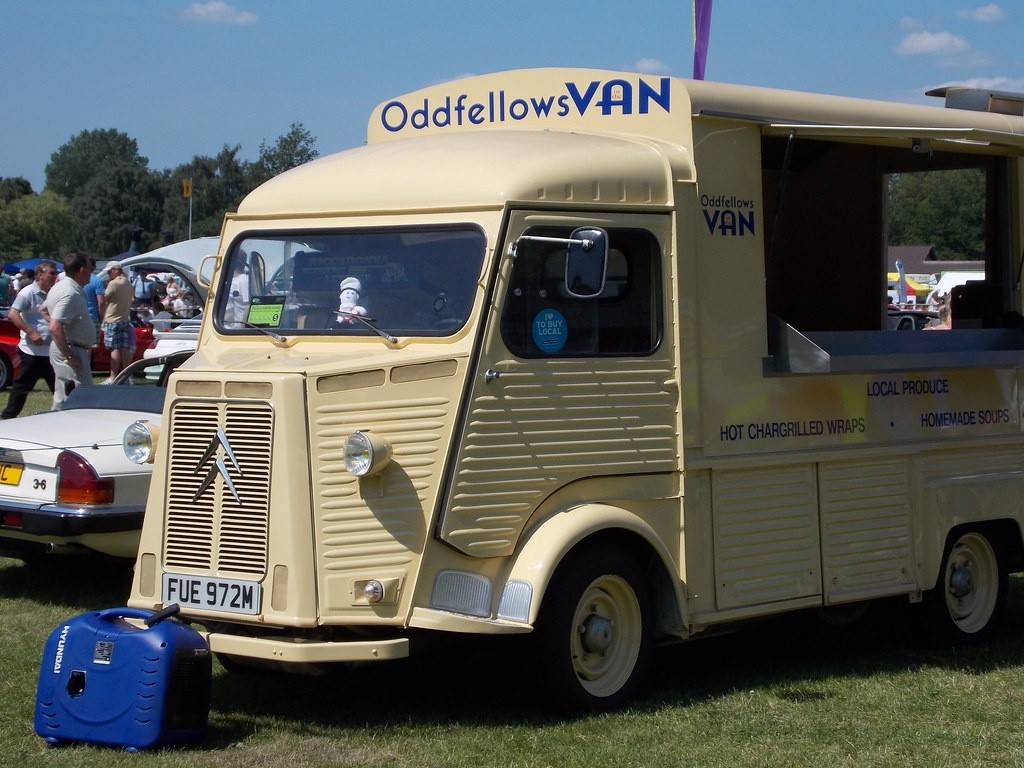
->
[335,277,367,324]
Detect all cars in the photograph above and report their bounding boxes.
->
[1,231,323,588]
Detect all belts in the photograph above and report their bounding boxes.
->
[66,342,92,351]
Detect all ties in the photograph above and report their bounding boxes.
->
[142,280,146,294]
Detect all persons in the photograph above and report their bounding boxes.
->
[38,248,96,411]
[223,248,249,329]
[920,291,952,330]
[83,261,194,386]
[0,260,58,419]
[0,264,35,306]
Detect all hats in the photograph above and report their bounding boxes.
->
[103,261,121,271]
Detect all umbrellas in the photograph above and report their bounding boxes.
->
[887,274,933,295]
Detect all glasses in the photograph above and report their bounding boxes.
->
[39,270,57,275]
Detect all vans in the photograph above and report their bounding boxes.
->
[115,65,1024,715]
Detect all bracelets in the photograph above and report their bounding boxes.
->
[66,353,73,360]
